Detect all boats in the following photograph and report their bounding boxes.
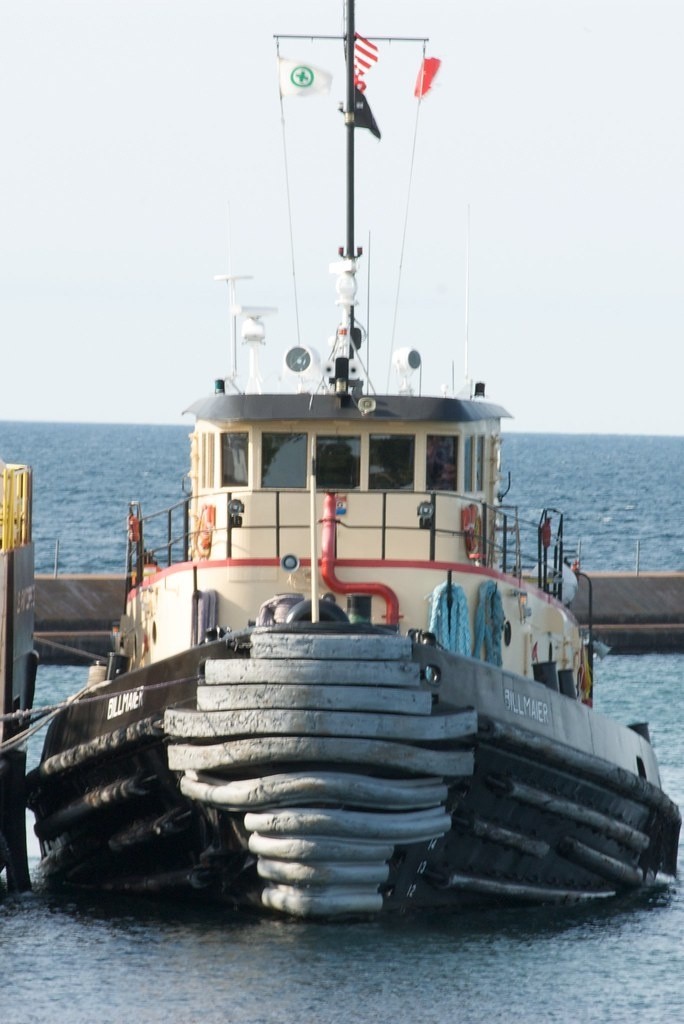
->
[17,0,684,928]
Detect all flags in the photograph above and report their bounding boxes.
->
[353,85,382,140]
[277,58,334,101]
[414,57,441,98]
[353,32,379,94]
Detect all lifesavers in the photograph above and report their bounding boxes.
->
[201,506,214,551]
[463,504,481,553]
